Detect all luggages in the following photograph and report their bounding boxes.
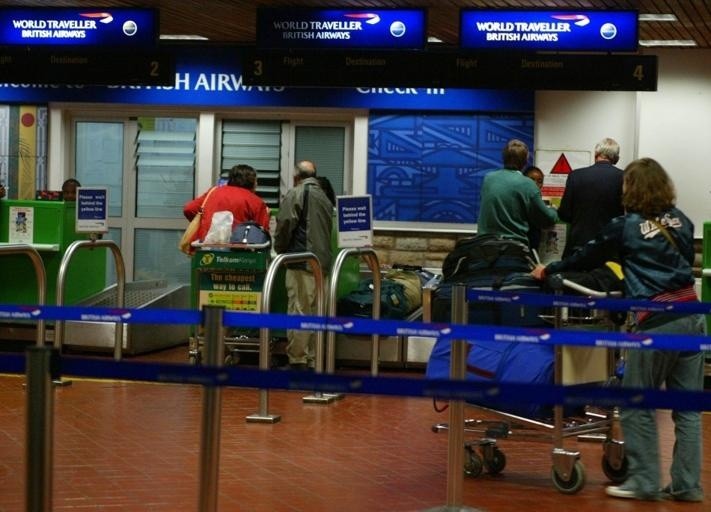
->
[433,284,545,328]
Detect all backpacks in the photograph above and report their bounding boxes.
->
[231,219,268,252]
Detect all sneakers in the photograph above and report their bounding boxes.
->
[657,484,705,502]
[604,481,660,501]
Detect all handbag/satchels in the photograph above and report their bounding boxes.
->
[338,264,435,322]
[424,320,587,416]
[442,232,539,281]
[178,212,202,258]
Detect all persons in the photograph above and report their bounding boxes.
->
[522,167,544,261]
[184,165,271,257]
[557,138,624,261]
[316,177,336,211]
[531,158,707,502]
[476,139,560,245]
[274,161,333,372]
[53,179,81,201]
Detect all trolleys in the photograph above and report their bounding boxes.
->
[186,236,287,370]
[423,280,631,494]
